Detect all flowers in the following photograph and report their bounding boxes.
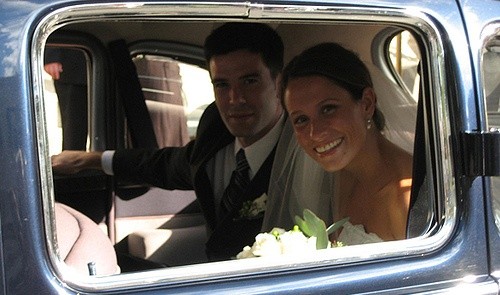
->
[236,208,351,259]
[232,192,267,225]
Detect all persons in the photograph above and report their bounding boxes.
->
[43,46,88,153]
[277,42,414,249]
[51,23,289,274]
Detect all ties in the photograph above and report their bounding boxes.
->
[218,148,251,220]
